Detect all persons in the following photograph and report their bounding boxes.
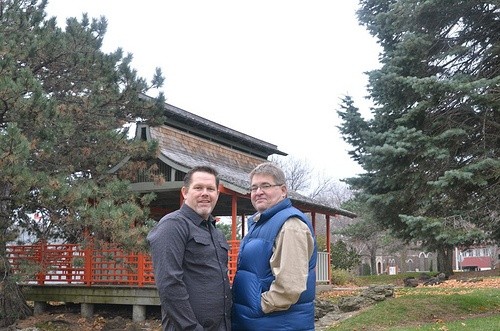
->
[144,166,232,331]
[230,162,317,331]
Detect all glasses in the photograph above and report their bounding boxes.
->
[247,183,283,192]
